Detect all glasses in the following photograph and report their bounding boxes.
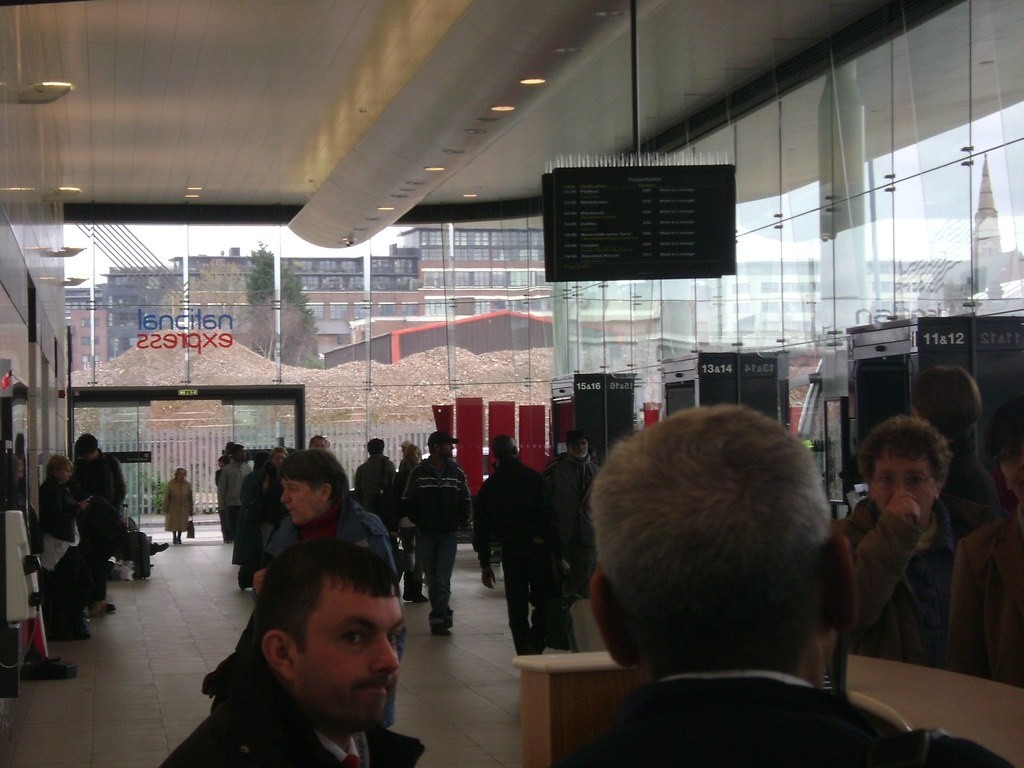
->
[869,474,932,492]
[992,443,1024,465]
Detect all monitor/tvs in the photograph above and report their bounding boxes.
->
[541,164,737,282]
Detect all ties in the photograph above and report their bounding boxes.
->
[341,755,361,768]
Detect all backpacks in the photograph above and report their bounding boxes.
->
[43,590,92,640]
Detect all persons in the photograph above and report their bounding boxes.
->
[251,449,400,628]
[164,468,194,546]
[157,540,426,768]
[216,430,601,654]
[828,364,1024,689]
[554,403,1013,768]
[68,433,168,615]
[39,454,93,644]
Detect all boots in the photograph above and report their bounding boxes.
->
[403,571,413,601]
[410,581,428,602]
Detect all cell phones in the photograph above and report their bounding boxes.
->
[82,496,93,504]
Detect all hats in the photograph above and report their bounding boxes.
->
[428,431,459,444]
[565,428,589,444]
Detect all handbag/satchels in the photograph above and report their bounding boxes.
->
[543,566,585,648]
[238,551,257,590]
[187,515,194,538]
[110,560,135,580]
[202,571,275,709]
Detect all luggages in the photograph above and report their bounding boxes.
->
[120,504,151,580]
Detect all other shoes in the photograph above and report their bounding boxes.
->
[431,623,451,635]
[445,606,453,628]
[173,537,182,545]
[390,528,401,538]
[398,516,416,528]
[149,541,169,556]
[106,603,115,612]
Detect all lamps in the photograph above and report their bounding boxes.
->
[17,81,72,104]
[41,187,80,204]
[63,277,88,286]
[55,247,86,258]
